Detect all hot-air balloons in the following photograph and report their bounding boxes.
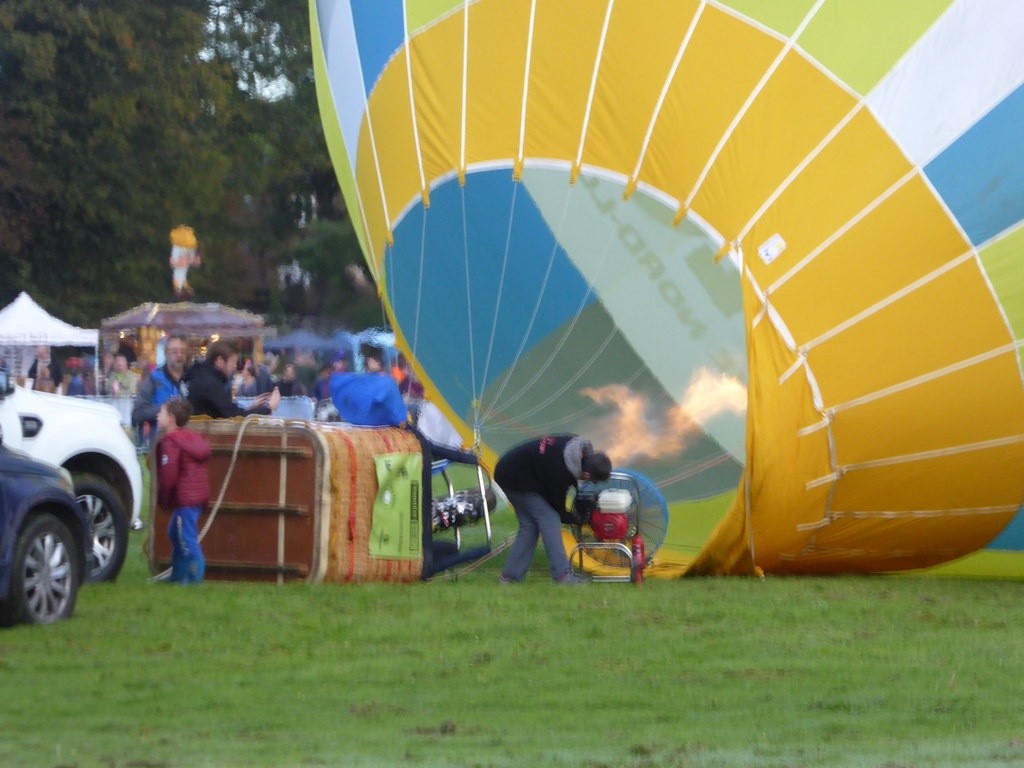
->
[142,0,1022,593]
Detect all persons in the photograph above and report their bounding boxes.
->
[20,327,389,593]
[493,432,613,586]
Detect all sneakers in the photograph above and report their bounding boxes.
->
[553,568,590,585]
[499,575,520,583]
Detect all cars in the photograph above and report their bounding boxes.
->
[1,356,151,585]
[0,436,93,630]
